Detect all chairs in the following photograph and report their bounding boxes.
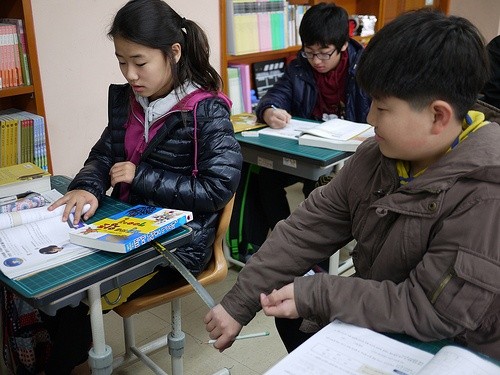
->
[112,192,236,375]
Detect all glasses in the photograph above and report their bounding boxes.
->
[300,44,338,60]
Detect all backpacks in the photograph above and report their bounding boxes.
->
[226,162,275,270]
[0,281,54,375]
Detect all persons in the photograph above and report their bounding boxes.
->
[204,6,500,365]
[46,0,244,375]
[257,3,374,277]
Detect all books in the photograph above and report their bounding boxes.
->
[232,0,311,56]
[226,62,252,116]
[70,204,193,254]
[0,18,32,89]
[259,318,500,375]
[0,108,104,281]
[256,119,376,152]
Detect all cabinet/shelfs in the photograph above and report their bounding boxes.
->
[0,1,54,177]
[220,0,382,115]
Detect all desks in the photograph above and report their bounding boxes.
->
[223,116,356,277]
[0,175,193,375]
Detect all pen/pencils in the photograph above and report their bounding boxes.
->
[204,332,269,344]
[271,105,290,128]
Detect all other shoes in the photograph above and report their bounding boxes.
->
[45,351,89,375]
